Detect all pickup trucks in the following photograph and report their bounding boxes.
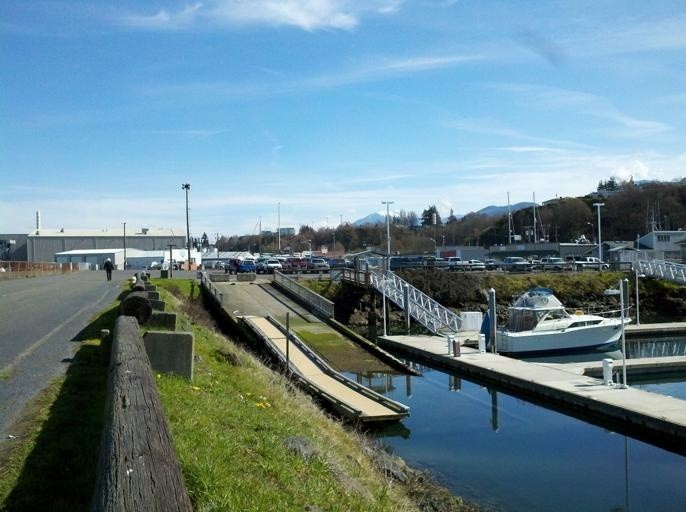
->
[568,256,609,270]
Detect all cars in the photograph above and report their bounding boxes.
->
[347,256,485,271]
[213,256,345,274]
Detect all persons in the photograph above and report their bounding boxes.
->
[102,258,114,281]
[130,273,137,289]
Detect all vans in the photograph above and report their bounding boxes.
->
[486,257,566,271]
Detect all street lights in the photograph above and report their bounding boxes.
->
[592,202,605,260]
[381,201,394,255]
[181,183,190,270]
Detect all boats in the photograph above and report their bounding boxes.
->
[495,287,633,353]
